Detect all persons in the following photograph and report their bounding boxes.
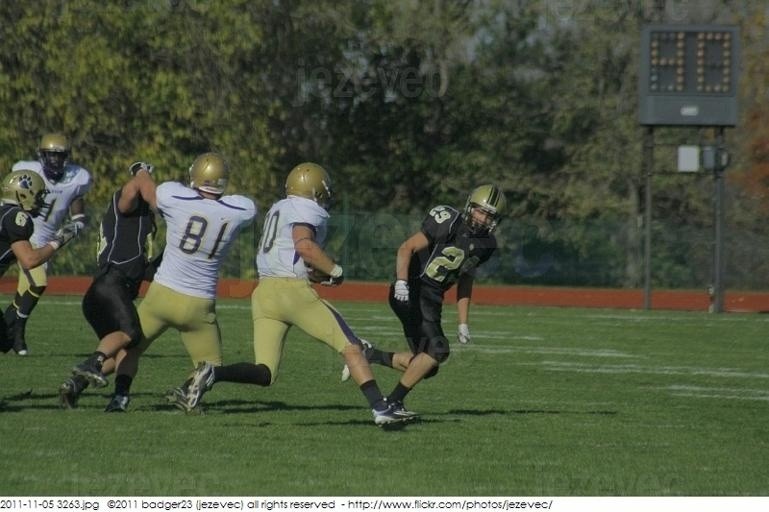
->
[60,163,159,410]
[0,169,89,353]
[1,132,91,356]
[186,162,422,426]
[343,185,508,424]
[107,152,257,412]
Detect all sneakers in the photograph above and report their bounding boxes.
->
[105,394,129,414]
[187,362,215,407]
[73,363,109,389]
[341,338,373,382]
[13,337,27,356]
[372,404,419,428]
[59,381,78,410]
[165,388,188,411]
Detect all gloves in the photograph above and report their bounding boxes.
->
[457,323,470,344]
[320,271,344,287]
[54,221,78,245]
[394,280,409,301]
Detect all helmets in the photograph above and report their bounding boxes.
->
[284,163,333,212]
[187,153,229,194]
[461,185,506,234]
[1,170,47,217]
[39,132,67,185]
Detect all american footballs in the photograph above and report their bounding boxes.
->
[306,264,344,288]
